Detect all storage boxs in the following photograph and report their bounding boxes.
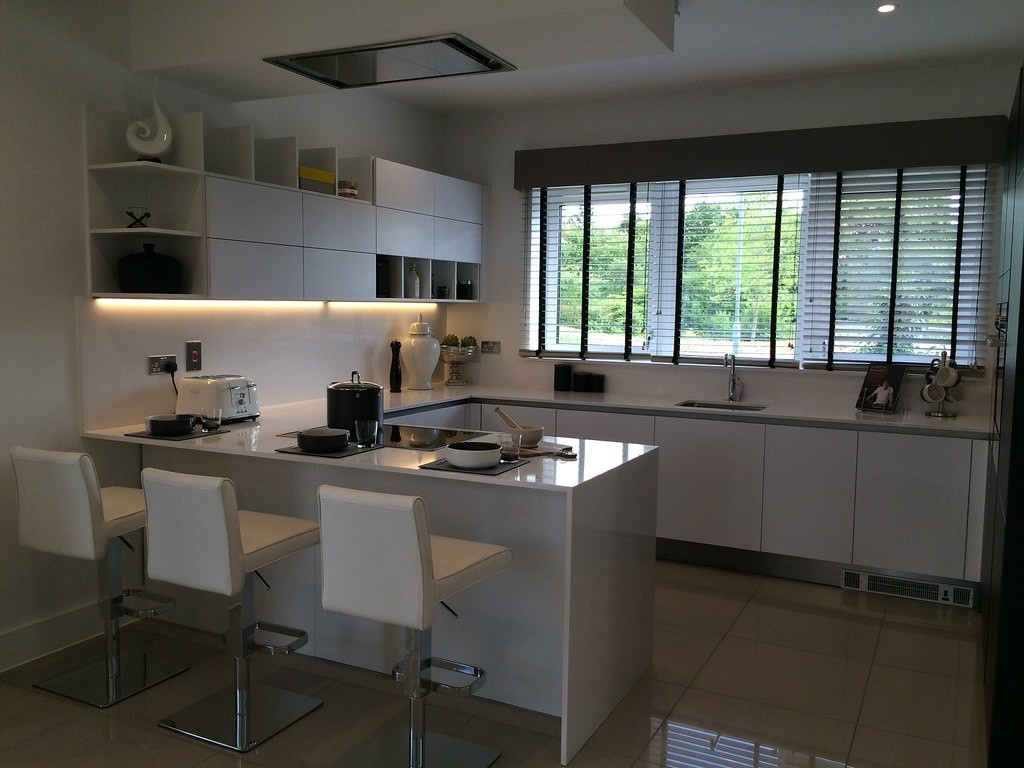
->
[299,165,335,195]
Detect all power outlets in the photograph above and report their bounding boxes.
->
[481,341,501,353]
[149,356,176,375]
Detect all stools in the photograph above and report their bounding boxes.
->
[316,483,514,768]
[141,467,329,753]
[11,444,189,707]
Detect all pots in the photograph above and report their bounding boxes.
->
[326,371,384,434]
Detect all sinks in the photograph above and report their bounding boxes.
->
[673,399,771,412]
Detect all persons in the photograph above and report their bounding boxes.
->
[866,379,893,408]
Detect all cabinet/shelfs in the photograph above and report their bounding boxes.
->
[81,61,1024,768]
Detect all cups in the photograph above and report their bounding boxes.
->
[355,419,378,449]
[437,282,449,299]
[944,382,969,403]
[496,433,522,464]
[200,408,223,433]
[935,366,961,388]
[921,374,946,404]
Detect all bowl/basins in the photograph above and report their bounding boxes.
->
[298,429,350,453]
[144,414,196,436]
[509,426,544,448]
[444,443,502,470]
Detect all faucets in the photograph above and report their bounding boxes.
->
[723,354,744,402]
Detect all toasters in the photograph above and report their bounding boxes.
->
[175,374,261,425]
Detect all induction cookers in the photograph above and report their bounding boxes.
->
[275,424,490,452]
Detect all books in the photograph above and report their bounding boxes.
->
[856,363,908,413]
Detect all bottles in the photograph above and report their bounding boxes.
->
[459,281,472,300]
[390,339,402,393]
[405,263,419,299]
[400,314,441,390]
[116,243,182,294]
